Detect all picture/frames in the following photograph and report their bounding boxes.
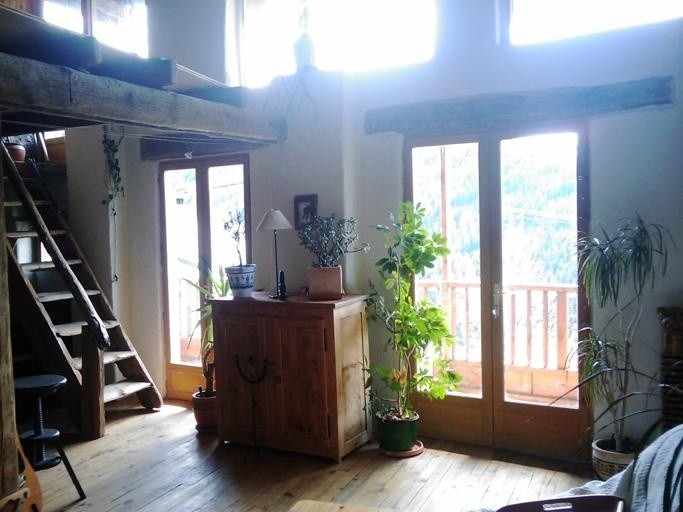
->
[293,193,318,230]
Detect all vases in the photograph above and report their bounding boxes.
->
[3,141,27,163]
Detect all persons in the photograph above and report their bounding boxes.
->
[301,203,309,221]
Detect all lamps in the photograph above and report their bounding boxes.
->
[255,209,294,301]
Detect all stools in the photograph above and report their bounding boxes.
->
[14,374,88,501]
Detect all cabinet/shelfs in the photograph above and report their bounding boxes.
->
[205,290,374,464]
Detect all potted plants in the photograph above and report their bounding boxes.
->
[562,211,675,482]
[177,252,231,439]
[298,210,372,303]
[223,201,257,300]
[359,200,464,458]
